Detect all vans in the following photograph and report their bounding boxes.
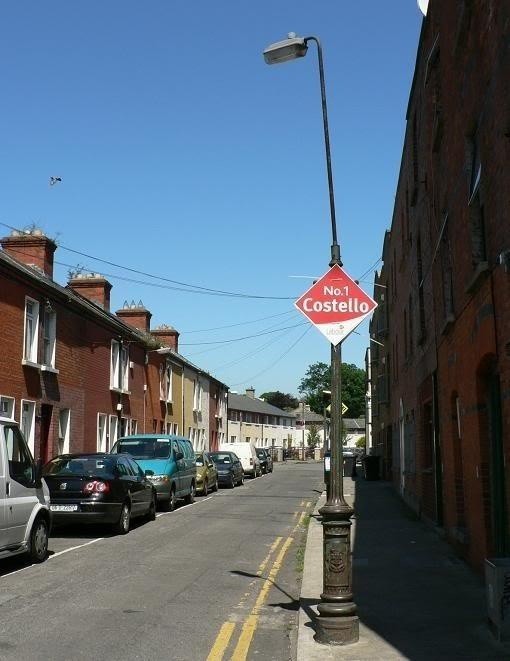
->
[107,432,199,515]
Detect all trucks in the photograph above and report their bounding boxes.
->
[1,414,55,569]
[217,441,264,478]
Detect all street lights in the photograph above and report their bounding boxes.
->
[248,23,372,646]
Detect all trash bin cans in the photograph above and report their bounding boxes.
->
[343,452,359,477]
[362,455,381,481]
[277,450,283,462]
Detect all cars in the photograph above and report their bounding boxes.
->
[39,448,158,537]
[353,446,366,464]
[193,445,276,497]
[341,445,354,455]
